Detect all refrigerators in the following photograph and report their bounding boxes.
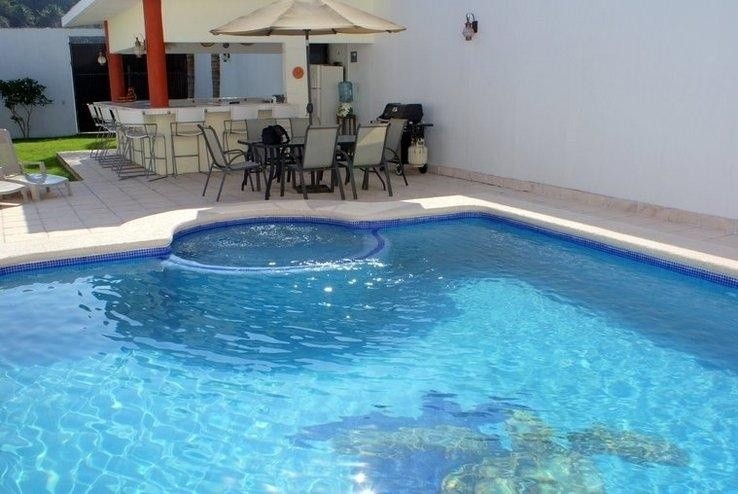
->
[308,63,345,129]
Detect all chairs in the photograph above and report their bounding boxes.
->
[1,128,73,204]
[197,116,407,203]
[86,103,271,182]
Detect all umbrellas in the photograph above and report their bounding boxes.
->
[209,0,407,185]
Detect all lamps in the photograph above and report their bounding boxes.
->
[96,38,109,65]
[462,11,477,41]
[133,32,147,58]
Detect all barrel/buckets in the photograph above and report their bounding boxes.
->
[338,81,353,103]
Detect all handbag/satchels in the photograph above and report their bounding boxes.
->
[408,135,428,168]
[262,125,291,144]
